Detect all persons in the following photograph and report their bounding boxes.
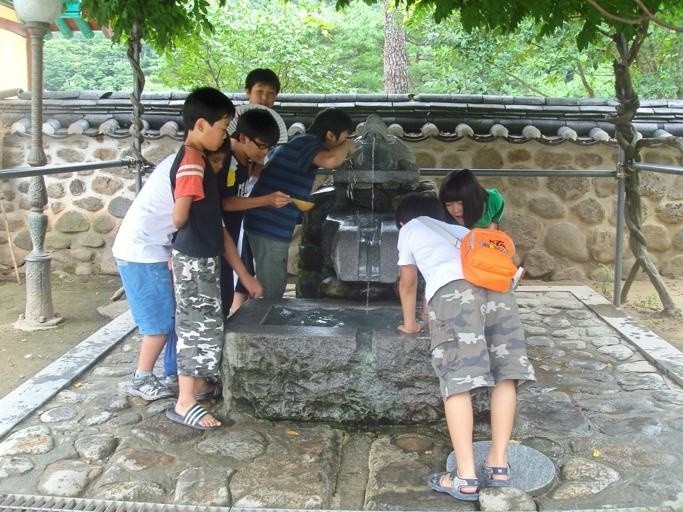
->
[222,109,290,315]
[167,88,236,432]
[229,70,288,290]
[243,108,356,300]
[111,129,231,402]
[394,195,534,500]
[439,170,506,229]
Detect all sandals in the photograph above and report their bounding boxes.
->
[428,469,480,501]
[481,459,512,487]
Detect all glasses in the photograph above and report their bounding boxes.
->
[251,139,275,151]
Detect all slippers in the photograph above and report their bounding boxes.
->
[166,404,221,430]
[195,382,220,400]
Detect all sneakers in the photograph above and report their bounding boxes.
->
[164,375,179,386]
[129,371,175,401]
